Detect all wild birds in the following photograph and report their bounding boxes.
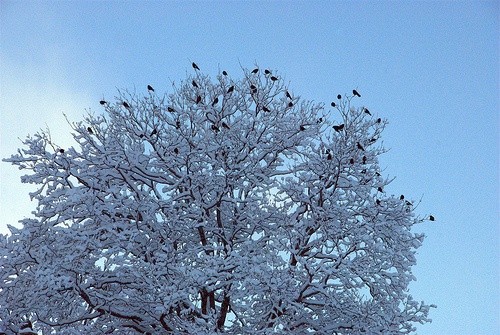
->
[284,89,436,222]
[100,100,106,104]
[263,106,270,112]
[265,69,278,81]
[222,70,228,75]
[227,86,234,94]
[212,98,218,106]
[124,101,129,108]
[192,80,198,87]
[196,95,201,104]
[168,107,175,112]
[150,128,157,137]
[192,62,200,70]
[148,85,154,91]
[60,149,64,153]
[222,122,230,129]
[252,68,258,74]
[211,124,220,132]
[88,127,93,134]
[250,84,257,94]
